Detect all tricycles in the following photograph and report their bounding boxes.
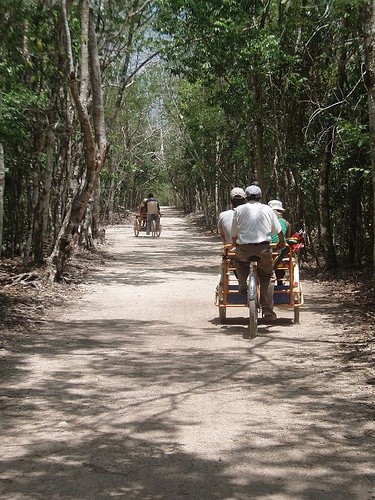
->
[214,237,305,339]
[134,211,164,238]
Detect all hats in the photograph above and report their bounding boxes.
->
[230,187,248,199]
[267,200,285,211]
[245,185,262,199]
[143,198,148,203]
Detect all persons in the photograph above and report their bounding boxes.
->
[267,200,291,284]
[141,198,148,229]
[231,185,276,320]
[144,193,161,235]
[219,187,247,278]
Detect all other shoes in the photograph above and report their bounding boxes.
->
[146,230,150,235]
[262,307,277,320]
[238,287,247,296]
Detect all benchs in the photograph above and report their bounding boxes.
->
[136,213,163,227]
[222,243,304,291]
